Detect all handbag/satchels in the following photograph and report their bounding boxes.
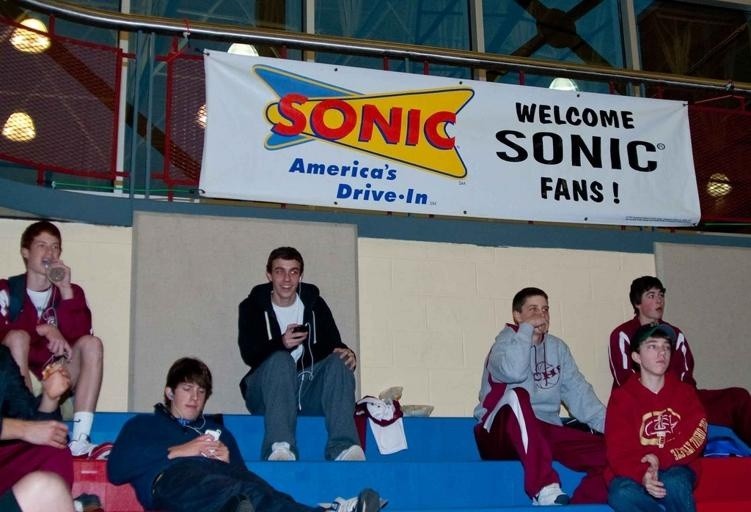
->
[71,441,145,512]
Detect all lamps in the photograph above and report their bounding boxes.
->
[198,103,207,129]
[549,78,580,91]
[707,171,731,197]
[11,12,49,52]
[0,98,35,143]
[225,41,260,59]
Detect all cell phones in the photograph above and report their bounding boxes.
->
[201,429,220,457]
[293,325,306,348]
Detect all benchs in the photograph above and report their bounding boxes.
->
[63,406,737,512]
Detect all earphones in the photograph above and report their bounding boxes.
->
[299,276,302,281]
[170,392,173,397]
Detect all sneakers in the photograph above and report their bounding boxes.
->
[317,487,390,512]
[267,441,297,461]
[334,444,366,461]
[67,433,99,457]
[531,482,570,507]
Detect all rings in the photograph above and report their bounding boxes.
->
[347,353,353,356]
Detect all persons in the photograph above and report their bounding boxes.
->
[0,222,115,461]
[106,356,389,512]
[474,287,610,506]
[238,247,366,463]
[1,344,75,496]
[1,471,78,511]
[608,275,751,452]
[601,321,709,511]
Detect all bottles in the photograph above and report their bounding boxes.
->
[45,257,65,284]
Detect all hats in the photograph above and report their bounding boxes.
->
[630,321,677,351]
